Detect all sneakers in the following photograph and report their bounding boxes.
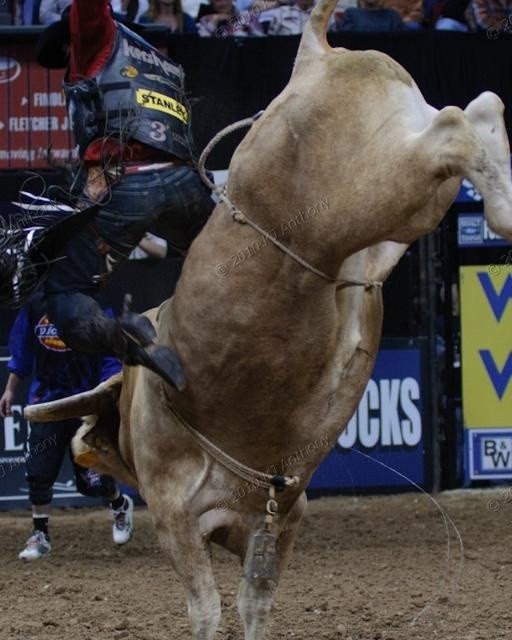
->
[111,493,136,548]
[18,529,52,562]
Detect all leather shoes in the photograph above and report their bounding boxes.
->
[114,316,186,394]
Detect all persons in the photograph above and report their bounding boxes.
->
[1,307,134,562]
[39,0,512,38]
[42,0,218,393]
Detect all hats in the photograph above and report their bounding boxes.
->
[34,4,145,70]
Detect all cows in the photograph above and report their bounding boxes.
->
[26,1,511,639]
